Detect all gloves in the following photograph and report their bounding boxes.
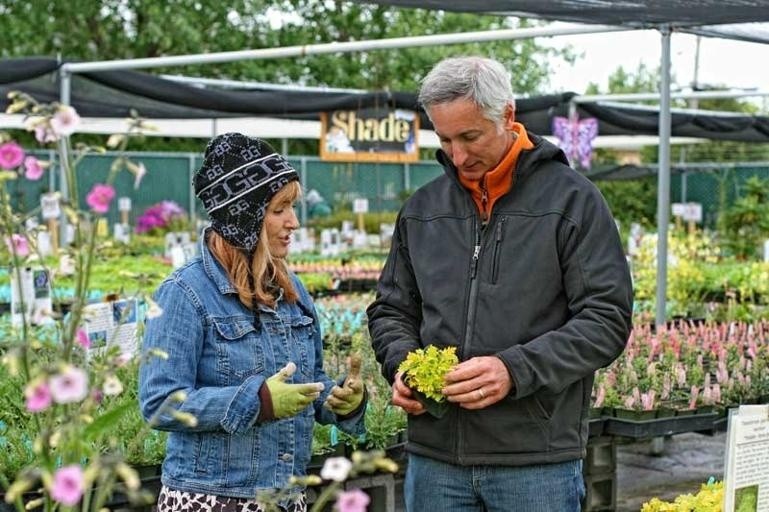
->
[257,357,364,423]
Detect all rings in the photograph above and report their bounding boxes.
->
[479,388,485,400]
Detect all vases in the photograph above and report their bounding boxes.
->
[588,405,713,420]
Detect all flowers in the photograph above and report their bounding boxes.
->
[0,90,459,511]
[589,318,769,409]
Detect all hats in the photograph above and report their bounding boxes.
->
[193,132,299,256]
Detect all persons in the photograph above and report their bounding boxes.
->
[364,52,635,512]
[137,128,369,512]
